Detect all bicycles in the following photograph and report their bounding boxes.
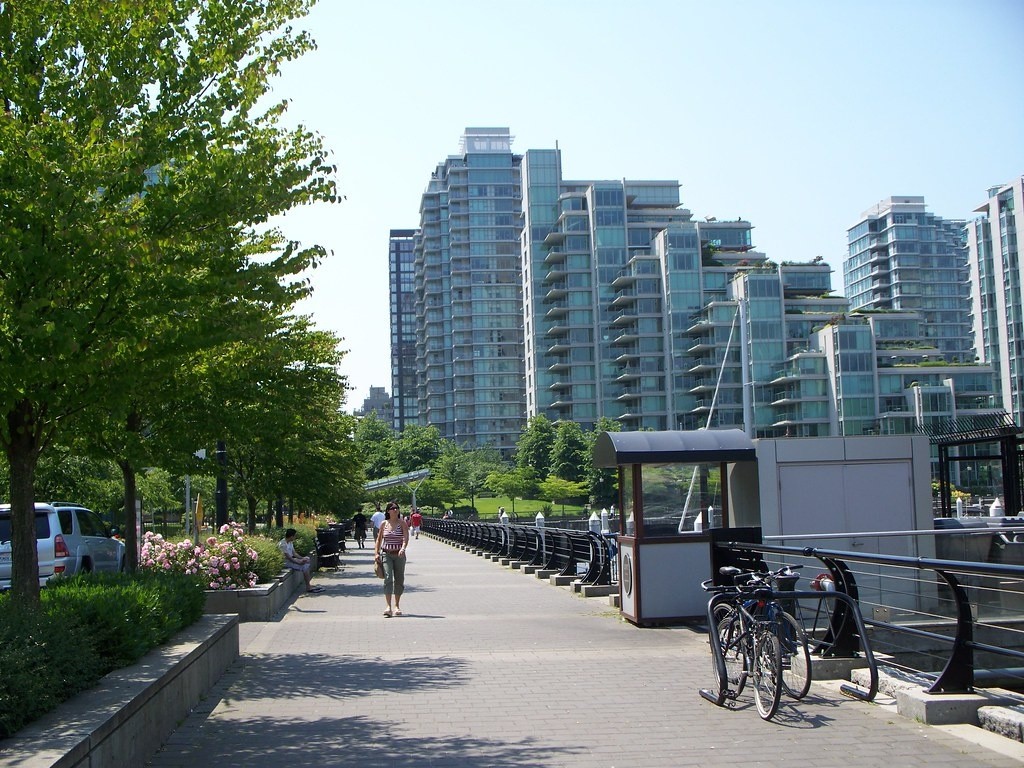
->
[700,563,814,722]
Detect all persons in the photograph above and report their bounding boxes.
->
[498,506,505,524]
[581,505,589,520]
[276,528,326,593]
[608,503,616,520]
[375,502,409,617]
[352,502,422,549]
[443,508,453,520]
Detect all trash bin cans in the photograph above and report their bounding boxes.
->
[342,519,352,537]
[315,528,341,567]
[328,523,346,550]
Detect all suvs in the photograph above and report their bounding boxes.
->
[0,501,127,589]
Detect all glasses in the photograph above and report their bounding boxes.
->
[389,506,399,510]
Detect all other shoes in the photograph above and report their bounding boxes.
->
[395,609,402,615]
[308,586,325,592]
[383,610,392,616]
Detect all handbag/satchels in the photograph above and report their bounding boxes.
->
[410,526,414,536]
[374,558,384,579]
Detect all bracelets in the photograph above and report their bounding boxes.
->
[401,548,405,551]
